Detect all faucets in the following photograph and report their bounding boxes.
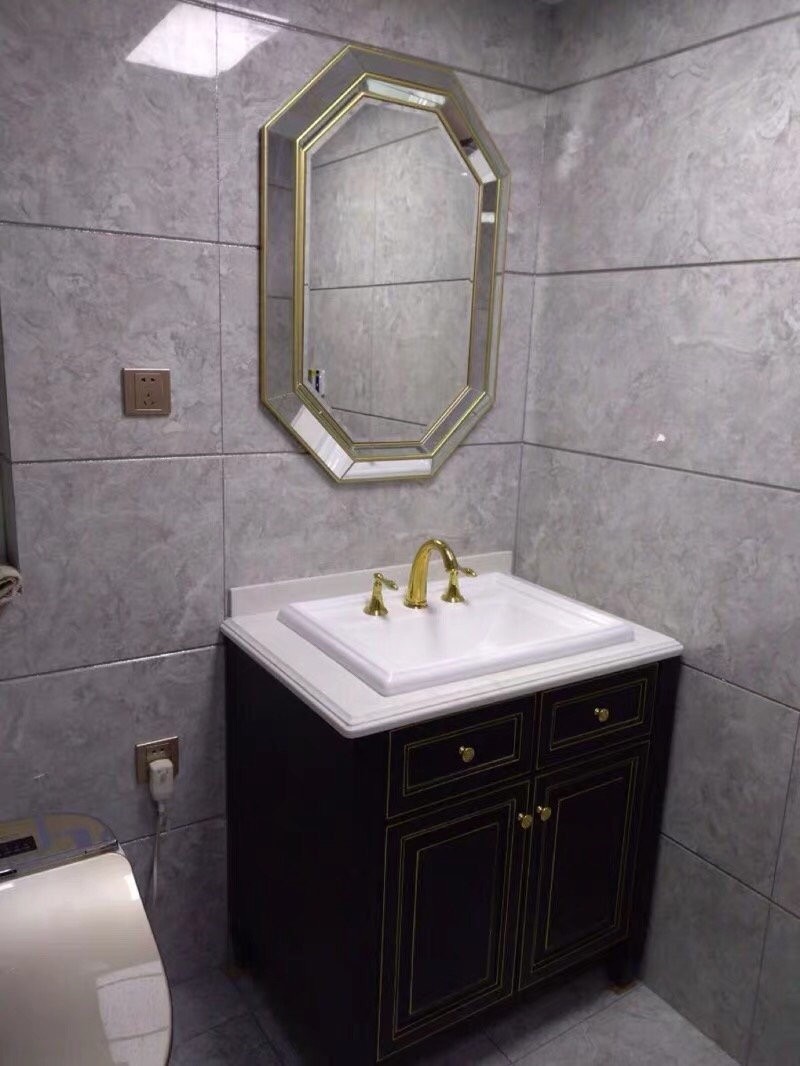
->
[404,537,460,610]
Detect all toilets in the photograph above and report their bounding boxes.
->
[0,810,176,1066]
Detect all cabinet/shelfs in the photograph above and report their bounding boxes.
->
[224,635,685,1066]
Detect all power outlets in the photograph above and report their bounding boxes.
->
[133,736,180,786]
[120,365,171,417]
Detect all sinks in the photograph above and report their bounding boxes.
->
[279,569,637,699]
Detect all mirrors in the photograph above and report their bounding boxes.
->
[256,40,513,483]
